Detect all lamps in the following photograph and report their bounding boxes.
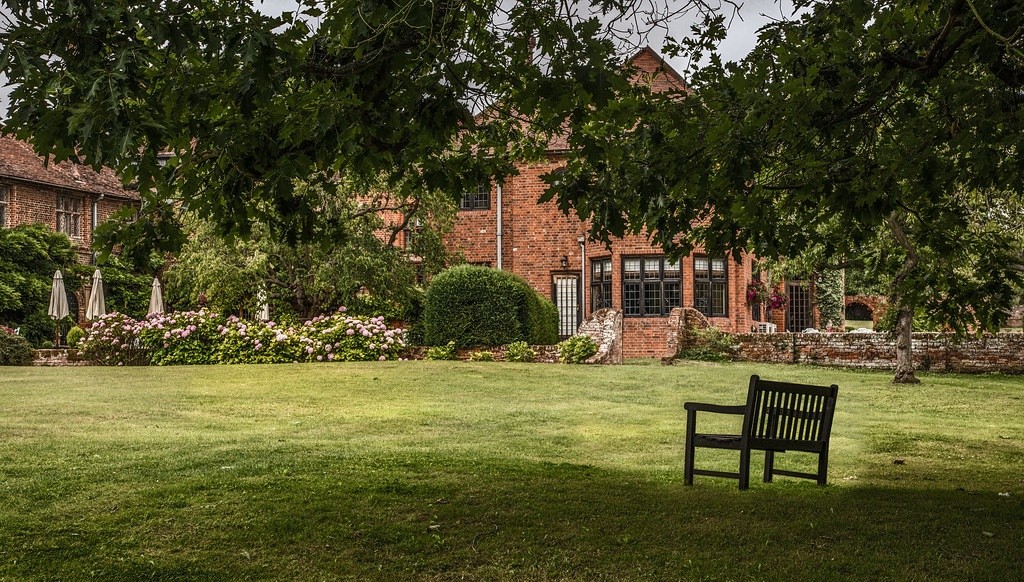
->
[561,256,568,269]
[817,273,823,283]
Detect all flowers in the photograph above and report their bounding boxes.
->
[747,280,789,312]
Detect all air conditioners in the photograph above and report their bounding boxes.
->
[759,322,778,333]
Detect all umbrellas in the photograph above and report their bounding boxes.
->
[86,269,106,323]
[254,278,270,326]
[48,270,69,348]
[148,276,166,318]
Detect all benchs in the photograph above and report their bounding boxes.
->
[682,375,839,492]
